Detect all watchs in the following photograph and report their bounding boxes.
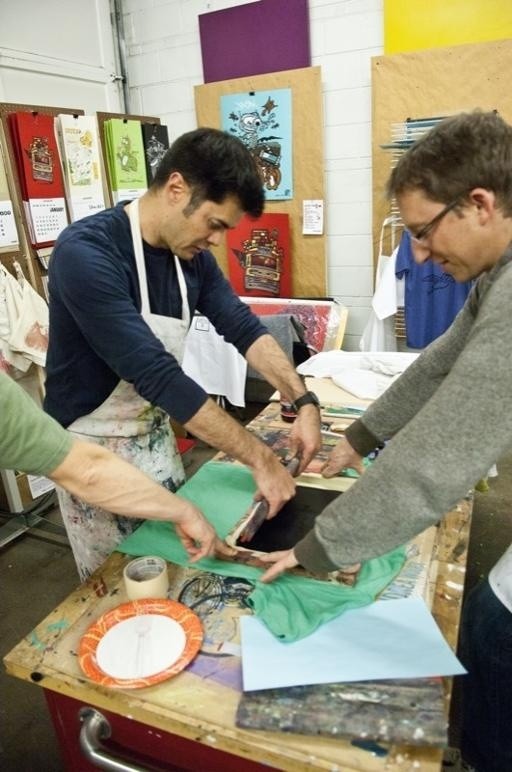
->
[288,390,321,414]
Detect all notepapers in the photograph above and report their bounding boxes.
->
[301,200,324,236]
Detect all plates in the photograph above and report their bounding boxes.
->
[77,597,205,691]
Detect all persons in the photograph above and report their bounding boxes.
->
[0,371,236,564]
[257,111,512,772]
[42,128,320,582]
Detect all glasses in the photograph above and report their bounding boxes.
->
[402,200,460,246]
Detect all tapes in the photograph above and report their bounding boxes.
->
[123,555,169,600]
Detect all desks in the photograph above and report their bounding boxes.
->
[3,351,475,772]
